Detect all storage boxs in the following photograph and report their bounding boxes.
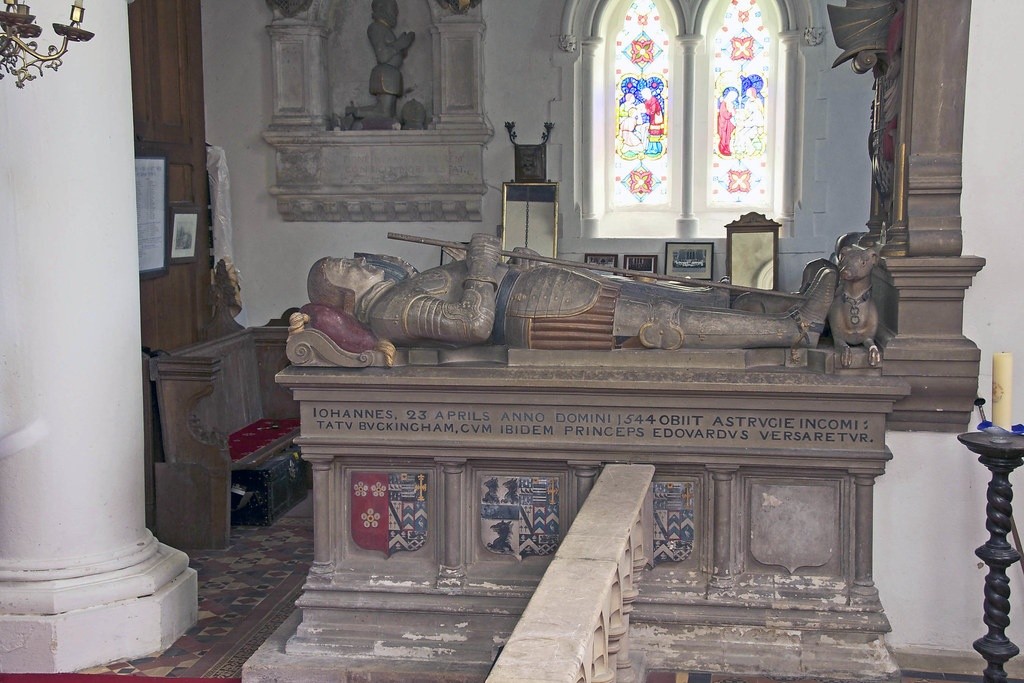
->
[231,450,308,527]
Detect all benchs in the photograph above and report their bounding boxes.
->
[227,417,300,478]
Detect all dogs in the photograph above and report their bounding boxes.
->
[829,244,882,368]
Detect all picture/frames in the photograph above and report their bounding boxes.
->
[168,206,200,263]
[623,254,658,277]
[664,241,714,280]
[585,253,618,275]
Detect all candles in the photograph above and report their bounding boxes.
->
[992,352,1014,431]
[74,0,83,7]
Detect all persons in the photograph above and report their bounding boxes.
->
[344,0,415,130]
[308,234,840,349]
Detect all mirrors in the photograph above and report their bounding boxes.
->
[724,212,782,291]
[502,182,559,262]
